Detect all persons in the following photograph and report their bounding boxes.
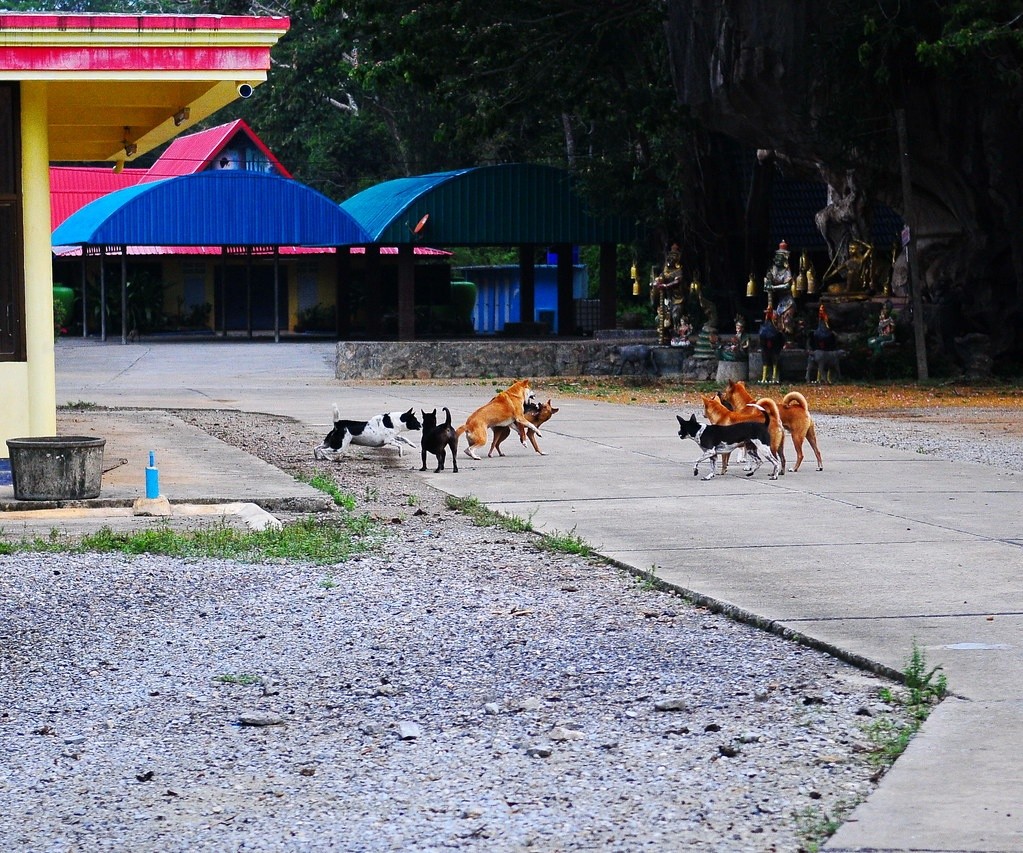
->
[653,247,684,330]
[866,300,895,346]
[829,237,872,292]
[765,242,793,301]
[715,320,750,361]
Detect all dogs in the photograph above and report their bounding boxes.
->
[314,403,422,461]
[700,395,787,476]
[487,398,559,458]
[419,407,459,473]
[455,378,543,460]
[675,404,782,480]
[723,379,824,472]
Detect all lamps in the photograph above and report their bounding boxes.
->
[172,107,191,126]
[121,139,137,157]
